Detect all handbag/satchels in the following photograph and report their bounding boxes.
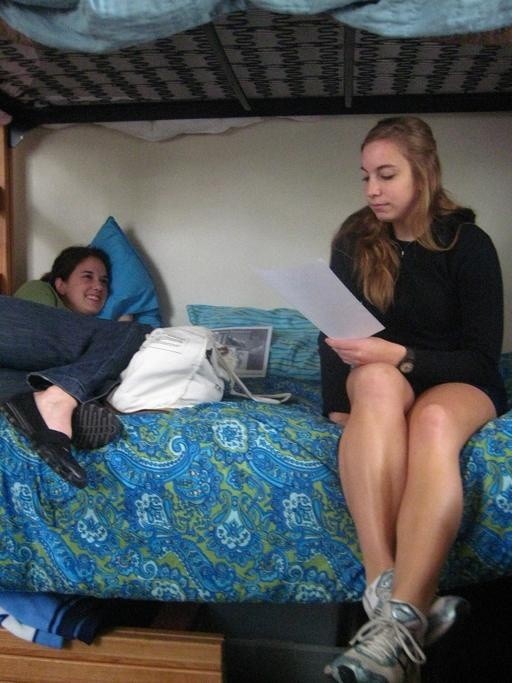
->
[103,325,225,414]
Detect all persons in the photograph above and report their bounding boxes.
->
[318,115,512,683]
[0,245,146,489]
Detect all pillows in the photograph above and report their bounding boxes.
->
[184,304,321,382]
[88,215,163,328]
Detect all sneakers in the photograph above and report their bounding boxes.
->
[1,389,123,488]
[323,567,467,682]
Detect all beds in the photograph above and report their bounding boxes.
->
[0,0,511,682]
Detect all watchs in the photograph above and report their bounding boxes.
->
[396,345,417,374]
